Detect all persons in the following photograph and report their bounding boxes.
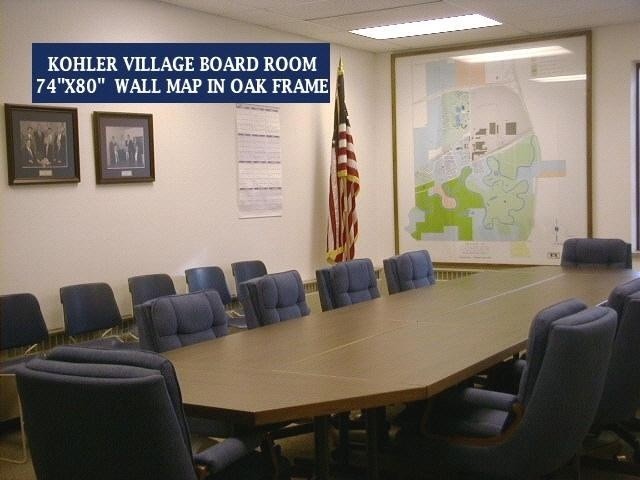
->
[107,132,145,170]
[19,123,69,168]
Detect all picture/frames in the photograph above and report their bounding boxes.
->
[2,101,82,186]
[91,110,156,186]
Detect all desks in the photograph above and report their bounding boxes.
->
[157,266,640,477]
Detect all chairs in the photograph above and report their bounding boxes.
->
[0,292,56,464]
[127,272,179,342]
[382,249,436,296]
[514,273,640,476]
[236,268,309,327]
[184,266,241,321]
[16,342,289,478]
[409,295,616,477]
[230,258,267,286]
[560,237,633,271]
[315,258,381,313]
[54,282,132,347]
[131,289,298,440]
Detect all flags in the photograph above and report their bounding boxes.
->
[322,67,370,266]
